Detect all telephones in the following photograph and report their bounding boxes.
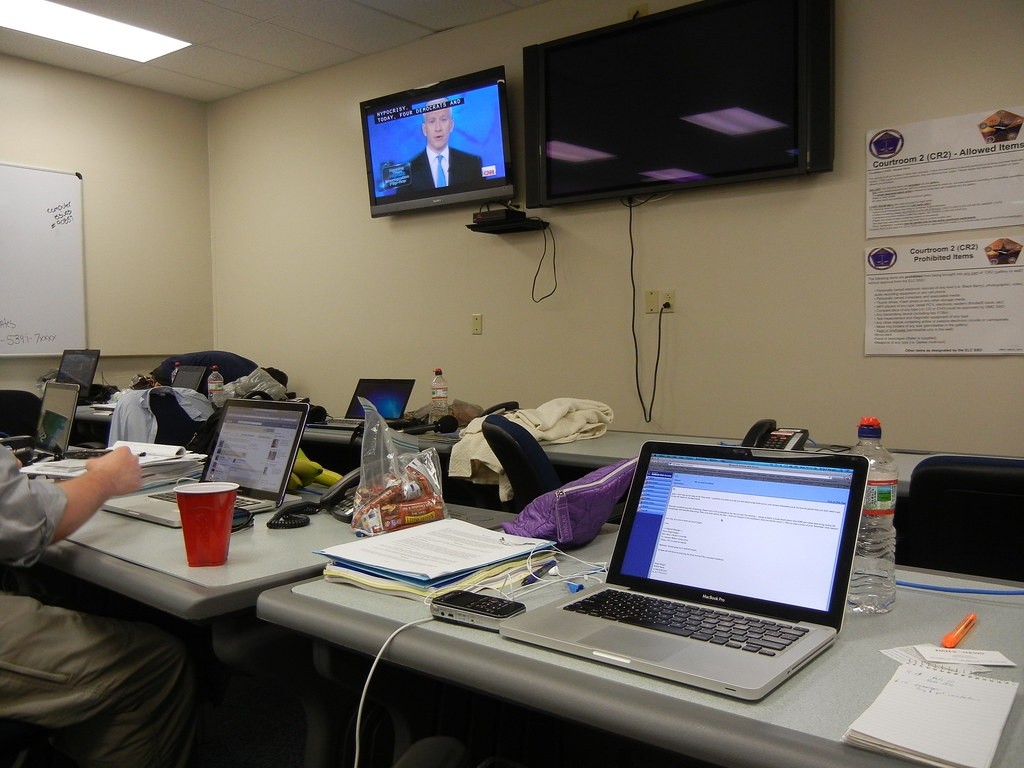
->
[481,400,519,416]
[742,417,811,454]
[320,458,411,525]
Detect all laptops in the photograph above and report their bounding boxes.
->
[171,365,206,391]
[54,349,101,406]
[100,397,311,528]
[31,382,80,465]
[501,440,870,701]
[307,378,415,430]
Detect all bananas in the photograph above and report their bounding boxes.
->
[286,448,343,490]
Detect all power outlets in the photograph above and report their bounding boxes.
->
[656,290,675,312]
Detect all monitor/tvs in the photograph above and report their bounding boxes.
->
[523,0,835,209]
[359,66,515,217]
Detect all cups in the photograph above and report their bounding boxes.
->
[173,482,240,567]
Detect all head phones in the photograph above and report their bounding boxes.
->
[305,405,328,424]
[404,415,458,435]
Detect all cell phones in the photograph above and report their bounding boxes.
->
[430,588,528,633]
[230,506,250,528]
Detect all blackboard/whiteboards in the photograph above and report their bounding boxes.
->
[1,161,89,358]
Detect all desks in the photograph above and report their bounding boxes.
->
[256,523,1023,768]
[38,484,520,768]
[74,406,110,423]
[299,427,930,499]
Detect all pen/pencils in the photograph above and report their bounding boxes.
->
[521,560,556,586]
[63,449,113,458]
[133,451,146,456]
[942,613,976,648]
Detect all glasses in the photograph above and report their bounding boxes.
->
[231,507,254,533]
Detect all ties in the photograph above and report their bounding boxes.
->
[436,155,446,188]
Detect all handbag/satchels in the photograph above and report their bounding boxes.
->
[502,455,639,552]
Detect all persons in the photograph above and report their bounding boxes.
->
[0,443,200,768]
[395,98,482,193]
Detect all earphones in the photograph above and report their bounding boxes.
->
[548,566,558,575]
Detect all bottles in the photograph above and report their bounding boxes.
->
[208,365,223,402]
[429,367,448,426]
[845,416,898,616]
[172,362,180,384]
[34,384,45,401]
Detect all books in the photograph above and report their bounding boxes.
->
[323,551,564,605]
[843,659,1019,768]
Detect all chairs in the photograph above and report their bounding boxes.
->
[907,454,1024,581]
[107,385,205,446]
[482,414,625,523]
[0,389,40,436]
